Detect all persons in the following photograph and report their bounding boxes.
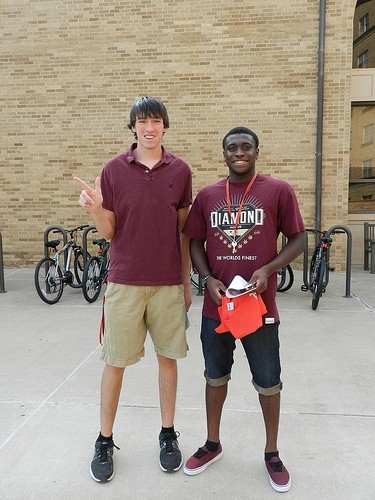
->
[73,96,192,483]
[181,126,306,492]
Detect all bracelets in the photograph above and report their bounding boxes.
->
[204,275,210,281]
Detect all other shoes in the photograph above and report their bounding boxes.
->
[183,442,223,476]
[265,457,290,492]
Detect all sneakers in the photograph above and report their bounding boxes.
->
[158,425,182,473]
[90,435,121,482]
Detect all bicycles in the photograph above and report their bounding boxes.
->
[34,224,96,305]
[82,230,112,303]
[276,251,295,292]
[190,267,207,292]
[304,228,346,310]
